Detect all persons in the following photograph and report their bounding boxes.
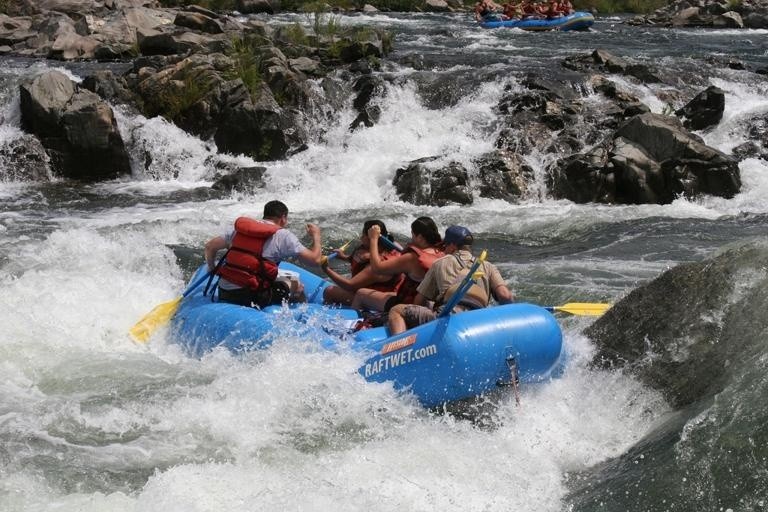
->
[386,225,515,337]
[322,219,400,309]
[350,215,447,311]
[475,1,576,24]
[202,198,323,310]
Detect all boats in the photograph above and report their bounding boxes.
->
[165,254,561,409]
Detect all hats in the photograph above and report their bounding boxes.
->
[435,225,473,246]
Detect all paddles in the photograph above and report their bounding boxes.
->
[128,259,226,344]
[544,303,614,316]
[321,238,353,263]
